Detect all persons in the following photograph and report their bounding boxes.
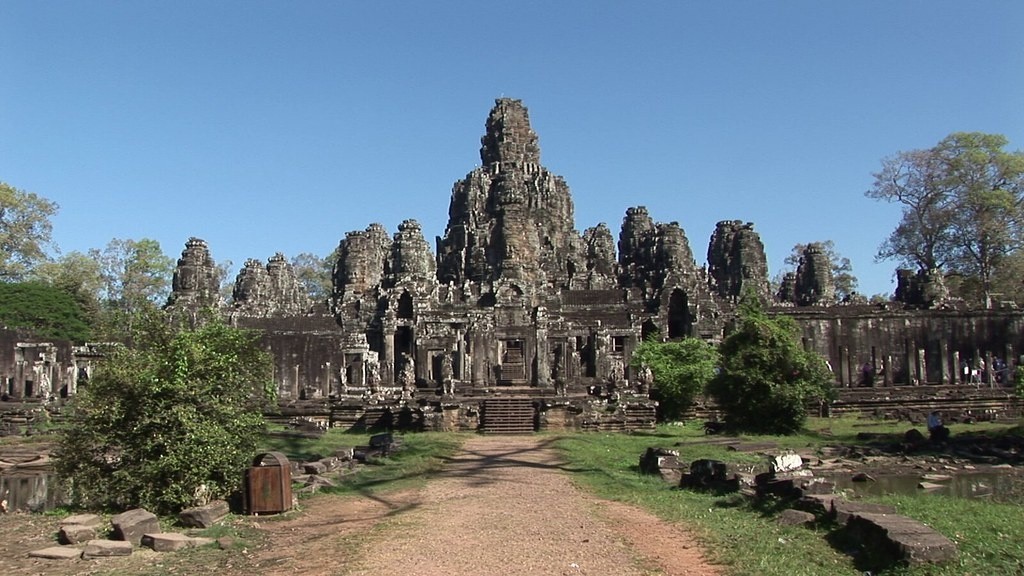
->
[863,363,871,385]
[961,356,1003,383]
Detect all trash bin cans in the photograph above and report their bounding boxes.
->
[242,452,292,514]
